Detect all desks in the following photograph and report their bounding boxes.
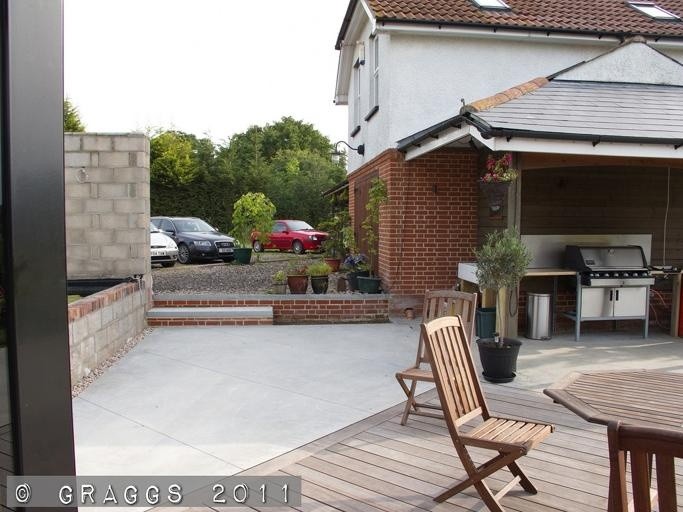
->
[457,263,581,343]
[543,367,683,487]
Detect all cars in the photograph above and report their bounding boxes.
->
[251,220,331,253]
[151,216,242,260]
[150,222,177,267]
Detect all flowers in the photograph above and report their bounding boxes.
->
[479,153,518,185]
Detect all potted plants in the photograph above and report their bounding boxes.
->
[232,177,388,294]
[472,227,534,383]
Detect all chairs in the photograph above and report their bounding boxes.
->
[420,314,556,512]
[395,290,478,426]
[607,421,683,512]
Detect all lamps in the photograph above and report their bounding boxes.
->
[328,141,364,164]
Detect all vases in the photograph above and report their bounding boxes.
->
[483,184,509,211]
